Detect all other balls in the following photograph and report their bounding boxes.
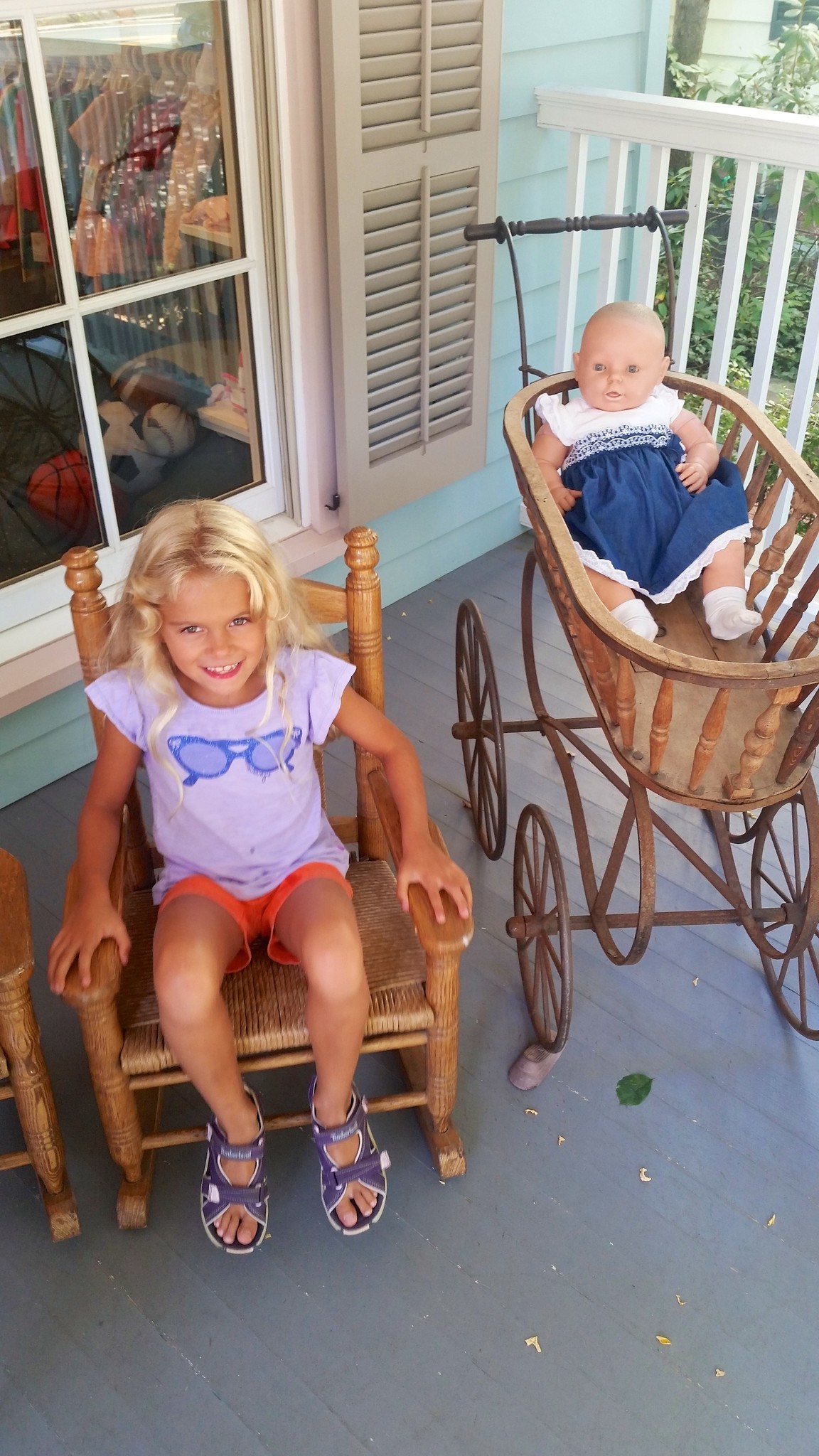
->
[143,403,195,457]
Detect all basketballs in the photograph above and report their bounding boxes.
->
[25,451,128,541]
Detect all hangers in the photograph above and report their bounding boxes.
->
[0,48,205,102]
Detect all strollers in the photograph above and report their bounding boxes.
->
[452,207,819,1049]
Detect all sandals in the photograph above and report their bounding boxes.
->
[200,1083,272,1255]
[307,1073,390,1237]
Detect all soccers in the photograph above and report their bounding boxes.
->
[79,397,170,495]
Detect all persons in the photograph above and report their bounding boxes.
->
[44,500,479,1254]
[534,301,763,644]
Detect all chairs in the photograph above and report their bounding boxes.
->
[0,843,84,1242]
[57,523,477,1230]
